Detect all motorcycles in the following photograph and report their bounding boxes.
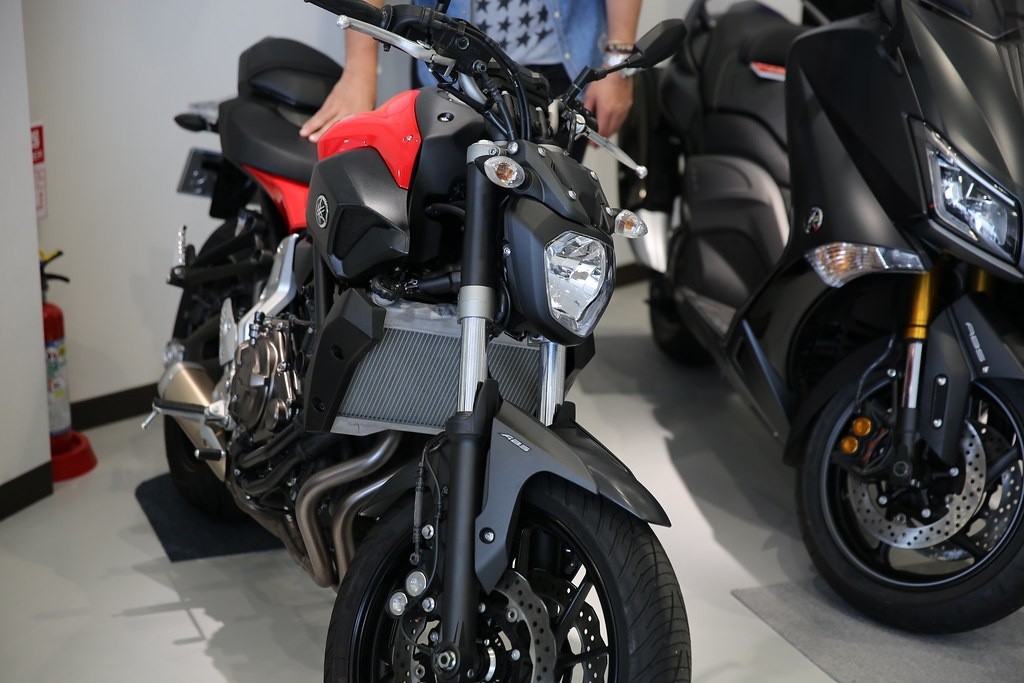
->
[618,0,1024,638]
[138,1,693,683]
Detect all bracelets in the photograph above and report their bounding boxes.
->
[603,41,635,53]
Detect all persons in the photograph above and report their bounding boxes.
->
[301,0,642,165]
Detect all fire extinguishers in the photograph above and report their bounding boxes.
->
[39,248,75,451]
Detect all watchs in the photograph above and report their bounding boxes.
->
[603,54,637,78]
